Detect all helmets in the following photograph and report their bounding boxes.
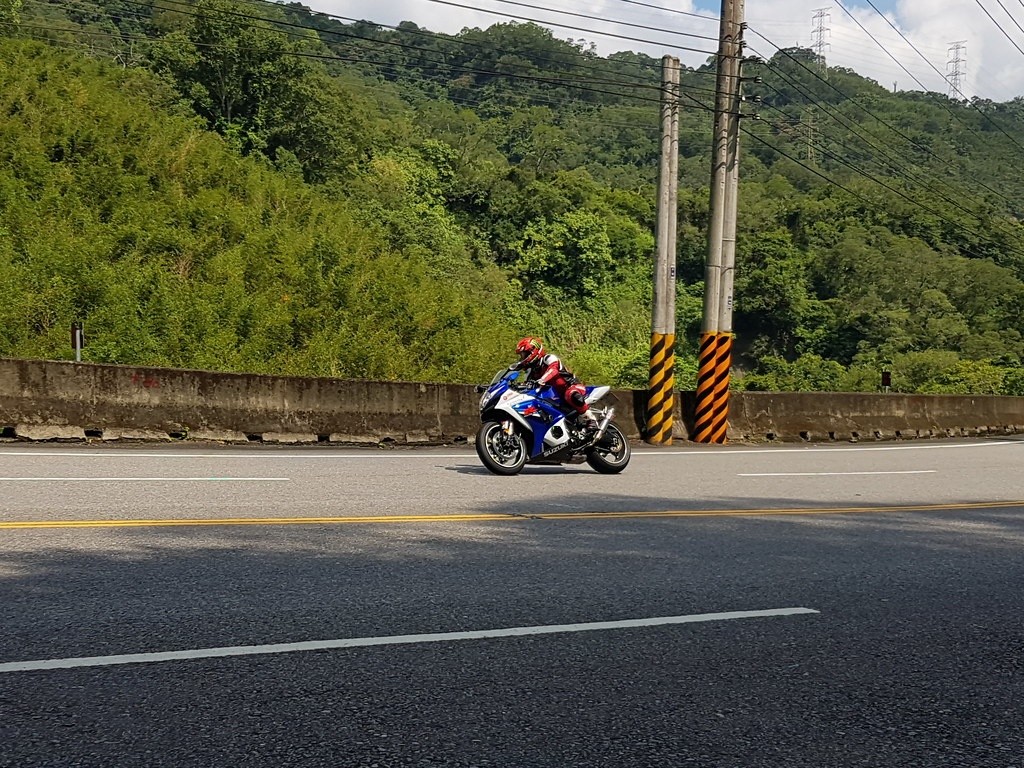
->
[516,336,546,367]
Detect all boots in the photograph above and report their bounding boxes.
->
[580,409,601,431]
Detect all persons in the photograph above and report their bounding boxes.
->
[515,336,601,430]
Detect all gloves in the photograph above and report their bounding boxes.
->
[526,379,544,390]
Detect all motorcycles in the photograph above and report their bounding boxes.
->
[474,362,632,475]
